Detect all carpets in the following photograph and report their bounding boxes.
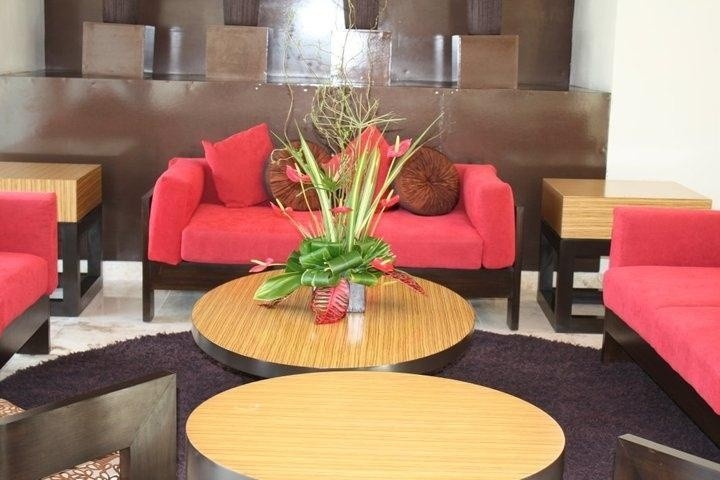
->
[0,327,719,480]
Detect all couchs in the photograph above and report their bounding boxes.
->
[141,157,526,333]
[601,207,720,448]
[0,192,57,370]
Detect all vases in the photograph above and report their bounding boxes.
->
[348,282,367,315]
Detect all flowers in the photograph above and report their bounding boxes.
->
[244,0,460,326]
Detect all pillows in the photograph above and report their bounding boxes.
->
[321,126,393,210]
[263,140,336,213]
[202,124,277,207]
[393,146,459,217]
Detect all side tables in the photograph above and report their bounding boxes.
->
[536,175,713,333]
[0,161,104,319]
